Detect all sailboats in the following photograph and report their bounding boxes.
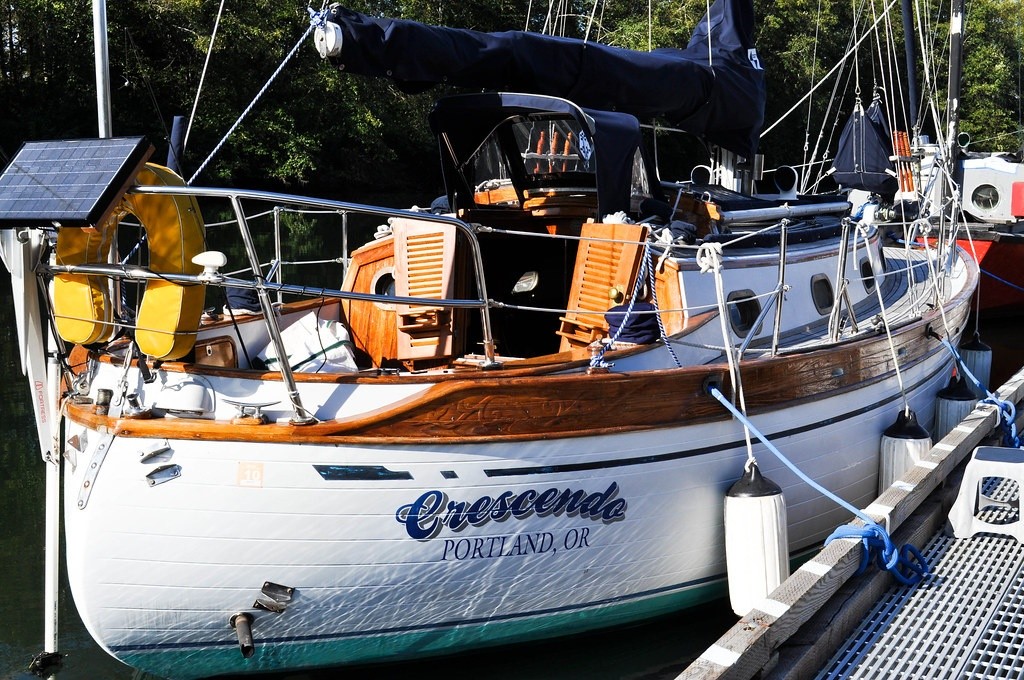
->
[0,0,1024,680]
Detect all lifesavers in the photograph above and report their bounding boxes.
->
[49,163,205,360]
